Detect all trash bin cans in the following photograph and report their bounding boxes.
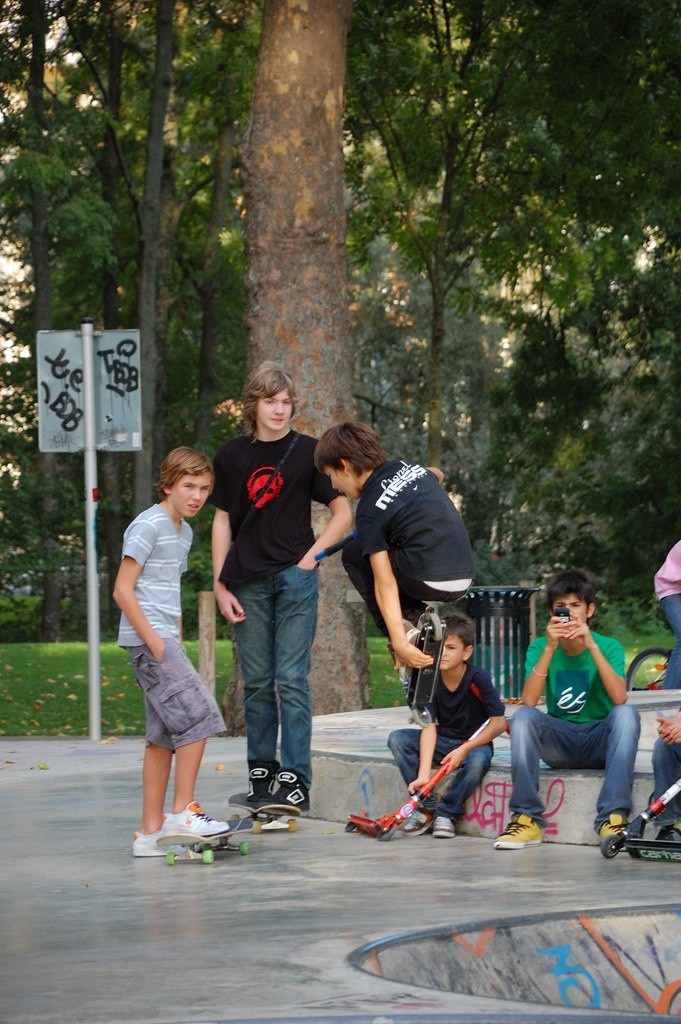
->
[454,586,541,700]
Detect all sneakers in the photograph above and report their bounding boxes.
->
[245,759,281,801]
[597,813,630,851]
[493,811,542,849]
[161,800,230,839]
[403,799,436,838]
[388,618,420,681]
[259,769,310,812]
[432,814,456,837]
[132,831,196,858]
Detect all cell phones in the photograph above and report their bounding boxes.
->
[555,607,571,630]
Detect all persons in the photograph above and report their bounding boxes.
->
[388,614,507,837]
[652,707,681,843]
[206,362,352,811]
[654,540,681,690]
[112,445,230,859]
[314,422,476,680]
[492,568,640,850]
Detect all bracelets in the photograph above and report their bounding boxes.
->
[532,667,547,677]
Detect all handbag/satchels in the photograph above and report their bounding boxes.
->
[218,543,239,586]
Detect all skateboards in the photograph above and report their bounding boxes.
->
[229,792,302,833]
[157,818,255,865]
